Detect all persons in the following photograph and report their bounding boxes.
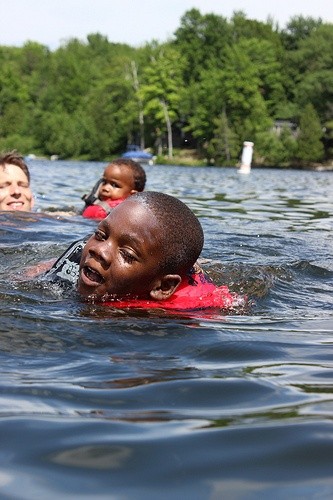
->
[0,148,32,213]
[82,159,146,220]
[24,191,232,321]
